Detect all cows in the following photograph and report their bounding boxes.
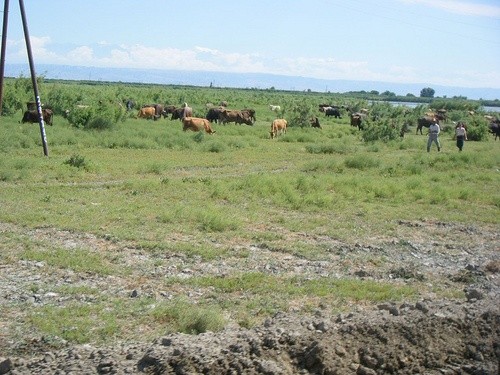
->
[22,109,54,126]
[126,100,499,139]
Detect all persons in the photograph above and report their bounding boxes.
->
[455,122,465,150]
[427,119,440,152]
